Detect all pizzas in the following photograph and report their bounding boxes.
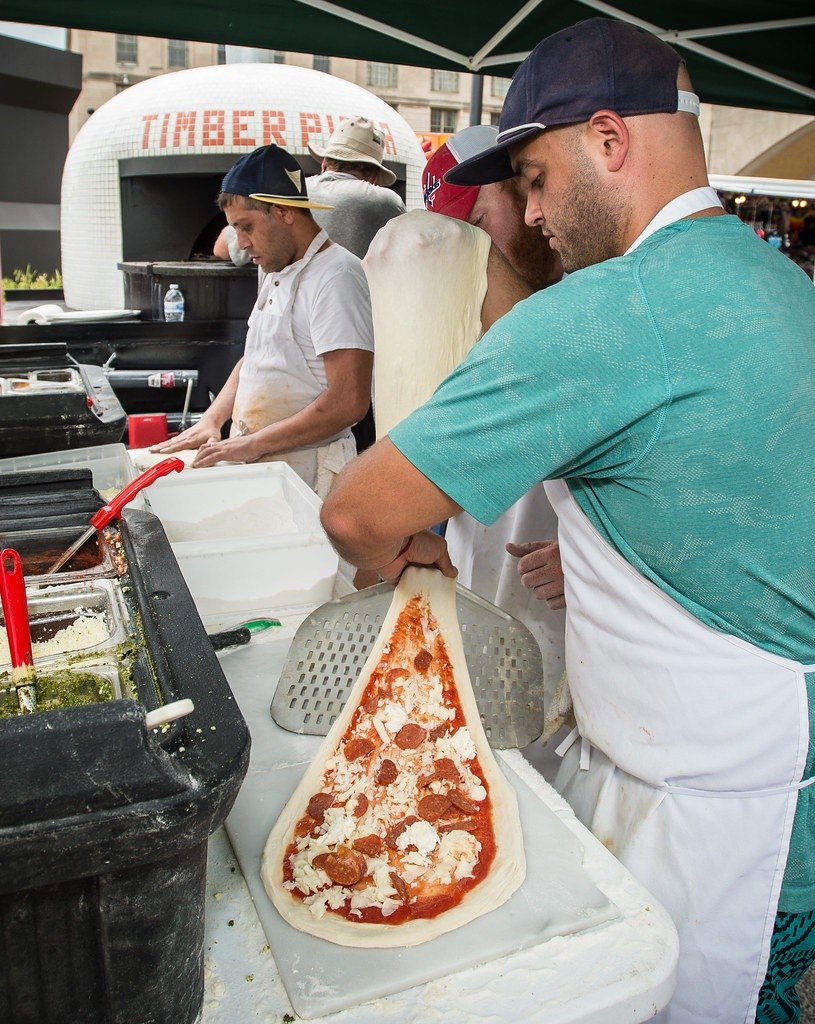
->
[260,558,528,948]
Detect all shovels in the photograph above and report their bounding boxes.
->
[269,513,546,748]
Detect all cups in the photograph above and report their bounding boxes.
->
[129,413,168,449]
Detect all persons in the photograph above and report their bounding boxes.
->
[149,118,585,786]
[321,19,814,1024]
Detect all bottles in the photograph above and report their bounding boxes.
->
[164,284,184,321]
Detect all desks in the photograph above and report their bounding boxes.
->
[132,444,679,1024]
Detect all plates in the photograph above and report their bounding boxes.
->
[44,309,141,321]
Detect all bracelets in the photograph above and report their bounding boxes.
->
[396,537,414,561]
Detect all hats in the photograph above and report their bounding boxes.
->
[421,125,499,221]
[221,144,335,210]
[306,115,396,187]
[443,15,700,187]
